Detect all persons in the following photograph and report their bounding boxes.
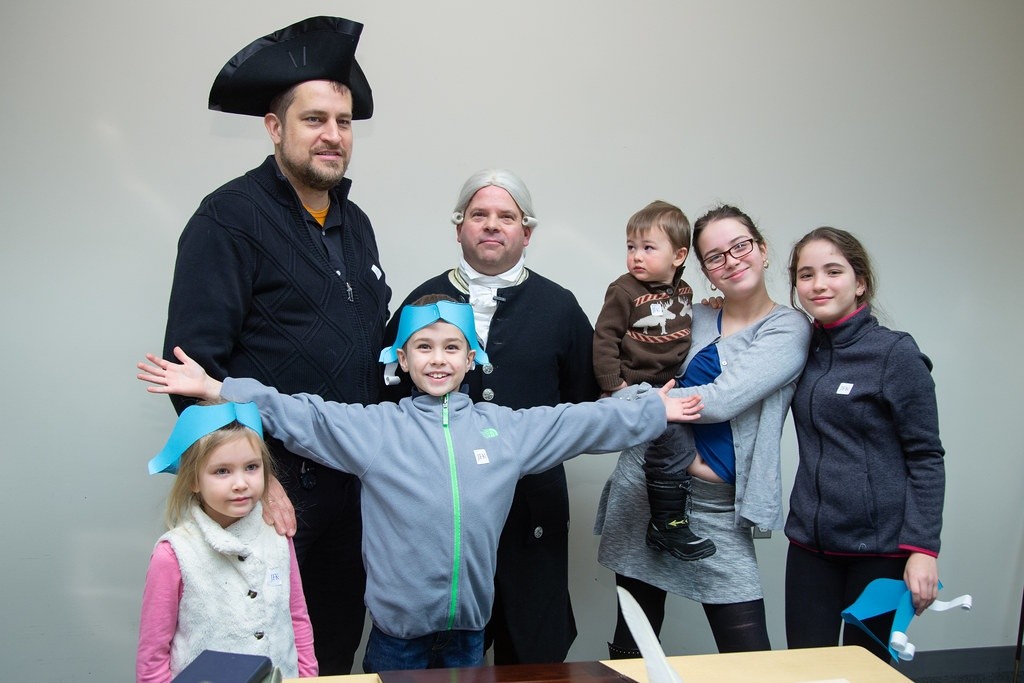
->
[377,162,603,665]
[700,225,947,665]
[135,395,321,682]
[161,14,393,678]
[591,198,722,563]
[133,288,706,675]
[592,203,815,661]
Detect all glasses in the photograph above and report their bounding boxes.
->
[703,238,759,271]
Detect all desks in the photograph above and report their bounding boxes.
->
[278,643,918,683]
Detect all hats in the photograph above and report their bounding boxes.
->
[208,16,373,120]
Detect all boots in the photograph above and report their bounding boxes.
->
[644,474,713,560]
